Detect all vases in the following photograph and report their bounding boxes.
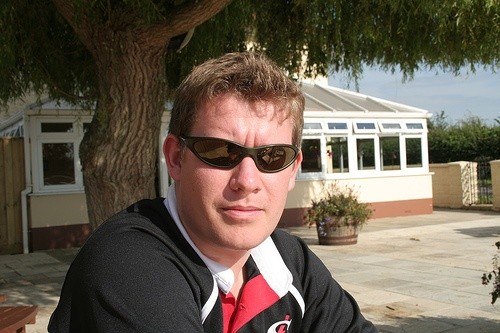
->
[315,219,361,245]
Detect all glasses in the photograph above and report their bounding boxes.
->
[179,134,300,172]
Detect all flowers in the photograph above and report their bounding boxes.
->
[299,185,374,237]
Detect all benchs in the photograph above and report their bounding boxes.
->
[0,303,39,333]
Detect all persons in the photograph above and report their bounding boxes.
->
[47,53,378,333]
